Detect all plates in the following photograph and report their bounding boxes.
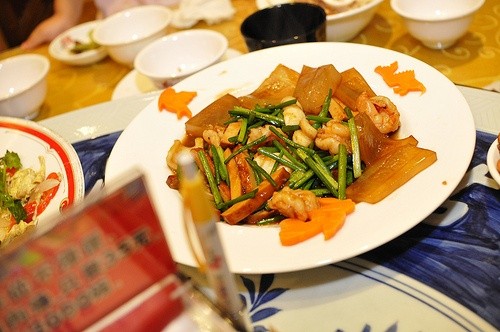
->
[180,258,500,332]
[104,43,478,275]
[111,49,242,102]
[0,116,85,250]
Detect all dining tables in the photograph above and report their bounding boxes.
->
[0,0,500,332]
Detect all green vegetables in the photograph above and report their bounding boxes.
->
[0,149,28,223]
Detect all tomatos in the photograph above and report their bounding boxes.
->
[11,172,59,228]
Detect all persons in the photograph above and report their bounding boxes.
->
[0,0,83,51]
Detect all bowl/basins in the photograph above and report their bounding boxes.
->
[91,4,173,70]
[389,0,485,50]
[0,53,50,121]
[487,137,500,186]
[255,0,384,43]
[133,29,229,89]
[48,19,107,66]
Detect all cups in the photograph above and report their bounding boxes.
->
[239,2,327,52]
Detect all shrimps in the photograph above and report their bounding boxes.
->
[355,89,402,135]
[202,127,223,149]
[290,116,318,149]
[315,120,355,156]
[165,135,203,173]
[248,123,284,152]
[263,184,318,223]
